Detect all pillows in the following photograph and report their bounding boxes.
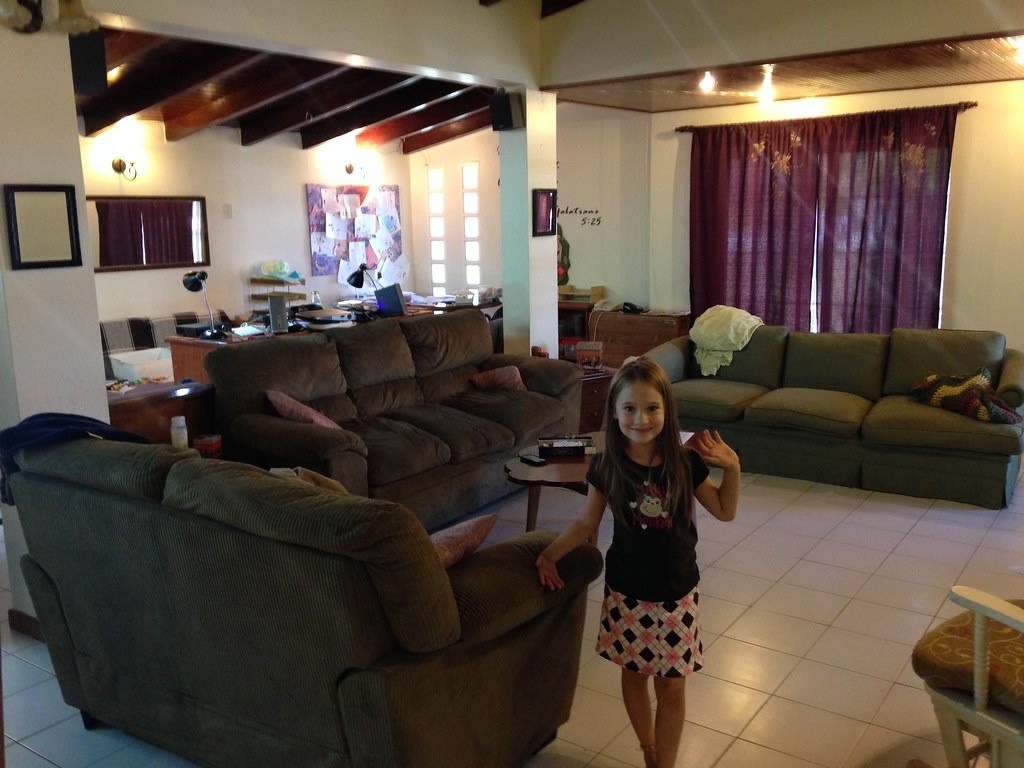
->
[428,514,498,568]
[470,364,528,393]
[266,389,342,429]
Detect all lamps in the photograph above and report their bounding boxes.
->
[0,0,101,35]
[346,262,389,319]
[182,269,230,342]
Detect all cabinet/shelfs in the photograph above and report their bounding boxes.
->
[578,366,613,434]
[588,310,691,371]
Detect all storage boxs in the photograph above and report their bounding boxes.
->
[108,347,173,382]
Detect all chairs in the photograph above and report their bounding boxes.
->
[912,586,1024,768]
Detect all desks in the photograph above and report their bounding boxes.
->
[162,308,435,383]
[557,301,594,340]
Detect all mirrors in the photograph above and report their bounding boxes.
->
[532,188,557,237]
[83,192,210,274]
[3,183,84,271]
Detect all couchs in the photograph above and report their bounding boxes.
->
[8,307,1024,768]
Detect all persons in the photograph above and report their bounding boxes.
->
[536,361,740,768]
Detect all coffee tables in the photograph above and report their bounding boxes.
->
[504,430,702,532]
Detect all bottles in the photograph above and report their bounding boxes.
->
[532,346,541,357]
[171,416,188,449]
[539,352,549,358]
[311,290,321,306]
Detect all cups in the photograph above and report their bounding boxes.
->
[268,295,289,335]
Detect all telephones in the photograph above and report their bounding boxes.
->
[593,299,622,312]
